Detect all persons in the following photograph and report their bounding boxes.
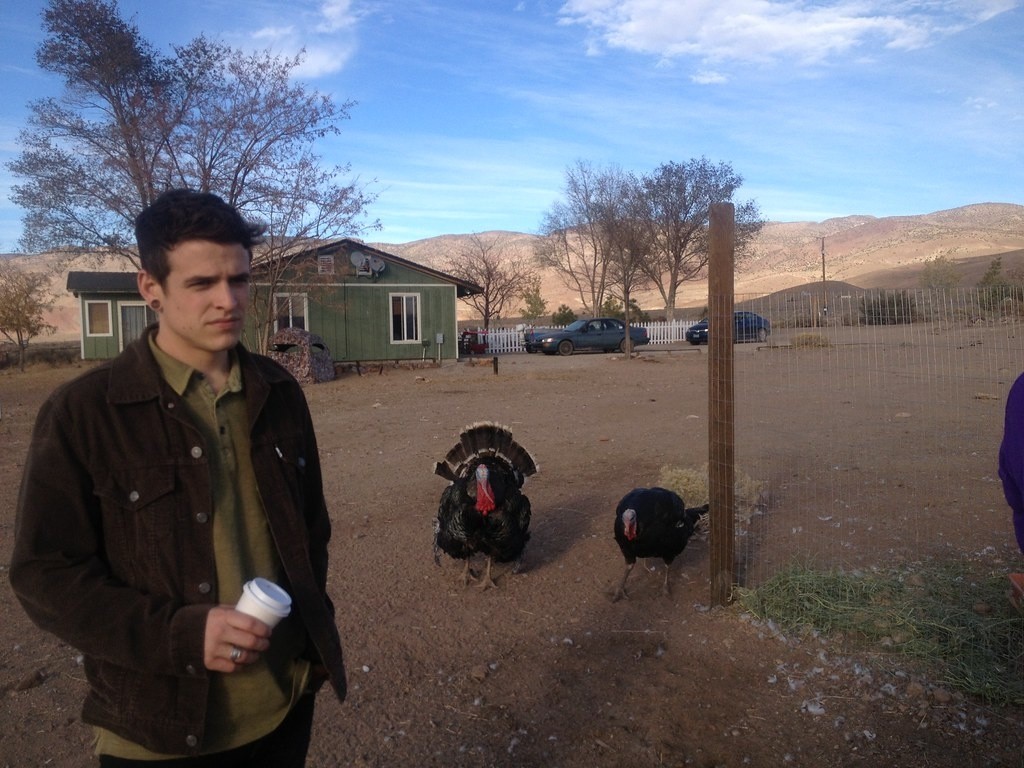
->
[10,189,347,768]
[998,373,1024,555]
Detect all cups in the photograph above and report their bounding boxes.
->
[235,578,291,628]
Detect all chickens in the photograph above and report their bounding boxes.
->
[601,485,710,604]
[431,420,538,592]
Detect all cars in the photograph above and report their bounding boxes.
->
[686,311,770,345]
[524,317,650,356]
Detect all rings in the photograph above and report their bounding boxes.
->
[230,645,242,662]
